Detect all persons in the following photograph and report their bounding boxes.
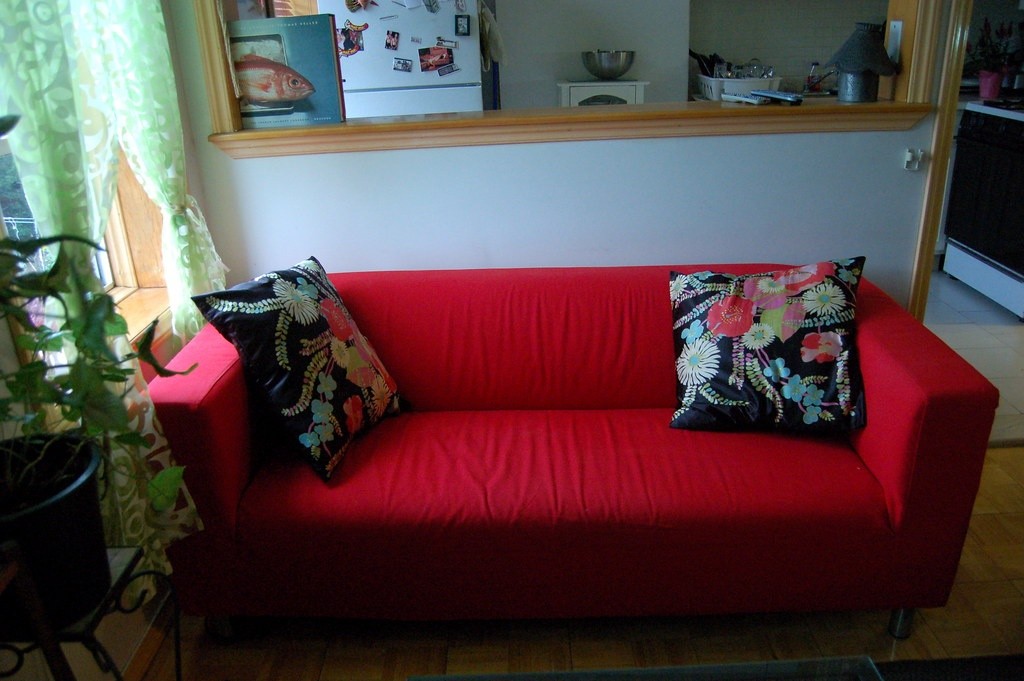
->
[385,32,409,71]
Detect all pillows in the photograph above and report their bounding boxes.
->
[668,256,866,427]
[189,255,412,484]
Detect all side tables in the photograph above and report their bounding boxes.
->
[0,547,183,681]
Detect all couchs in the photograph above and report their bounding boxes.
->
[142,264,998,637]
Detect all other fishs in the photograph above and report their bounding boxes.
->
[233,54,316,101]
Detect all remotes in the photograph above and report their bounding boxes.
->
[750,90,803,105]
[721,92,772,106]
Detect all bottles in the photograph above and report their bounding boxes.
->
[807,62,822,92]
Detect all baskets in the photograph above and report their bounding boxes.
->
[696,73,783,100]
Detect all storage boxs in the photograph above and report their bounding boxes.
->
[697,73,784,100]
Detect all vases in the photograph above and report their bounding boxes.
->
[978,71,1002,99]
[0,112,197,633]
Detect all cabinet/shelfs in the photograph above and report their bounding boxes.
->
[933,108,1024,322]
[555,81,652,107]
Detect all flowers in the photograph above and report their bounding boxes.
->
[964,15,1018,77]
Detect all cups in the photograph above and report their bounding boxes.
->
[713,61,775,79]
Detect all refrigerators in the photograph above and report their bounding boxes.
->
[319,0,483,119]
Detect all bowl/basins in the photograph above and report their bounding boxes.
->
[582,51,634,79]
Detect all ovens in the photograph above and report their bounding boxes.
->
[944,128,1024,282]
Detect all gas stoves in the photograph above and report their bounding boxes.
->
[959,89,1024,148]
